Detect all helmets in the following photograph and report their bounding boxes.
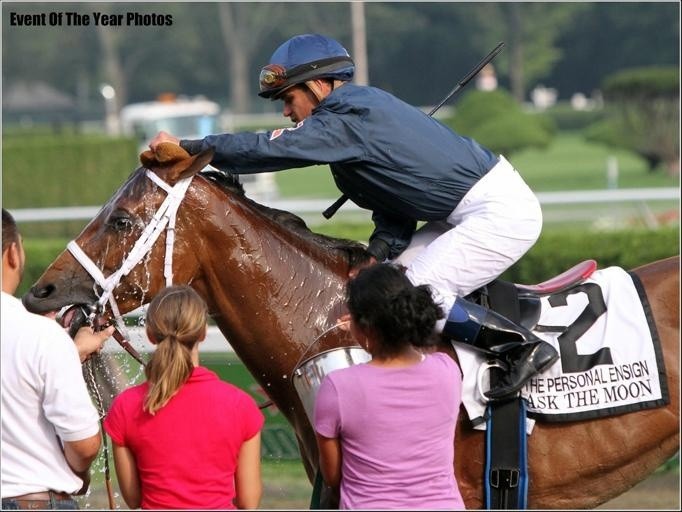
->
[258,33,355,100]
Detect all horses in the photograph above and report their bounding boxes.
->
[22,145,680,511]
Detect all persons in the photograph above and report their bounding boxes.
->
[1,207,117,511]
[308,261,466,510]
[148,33,560,402]
[102,284,264,510]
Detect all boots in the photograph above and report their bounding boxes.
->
[442,294,559,398]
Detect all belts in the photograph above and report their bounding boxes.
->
[11,492,69,500]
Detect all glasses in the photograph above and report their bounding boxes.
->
[257,64,287,91]
